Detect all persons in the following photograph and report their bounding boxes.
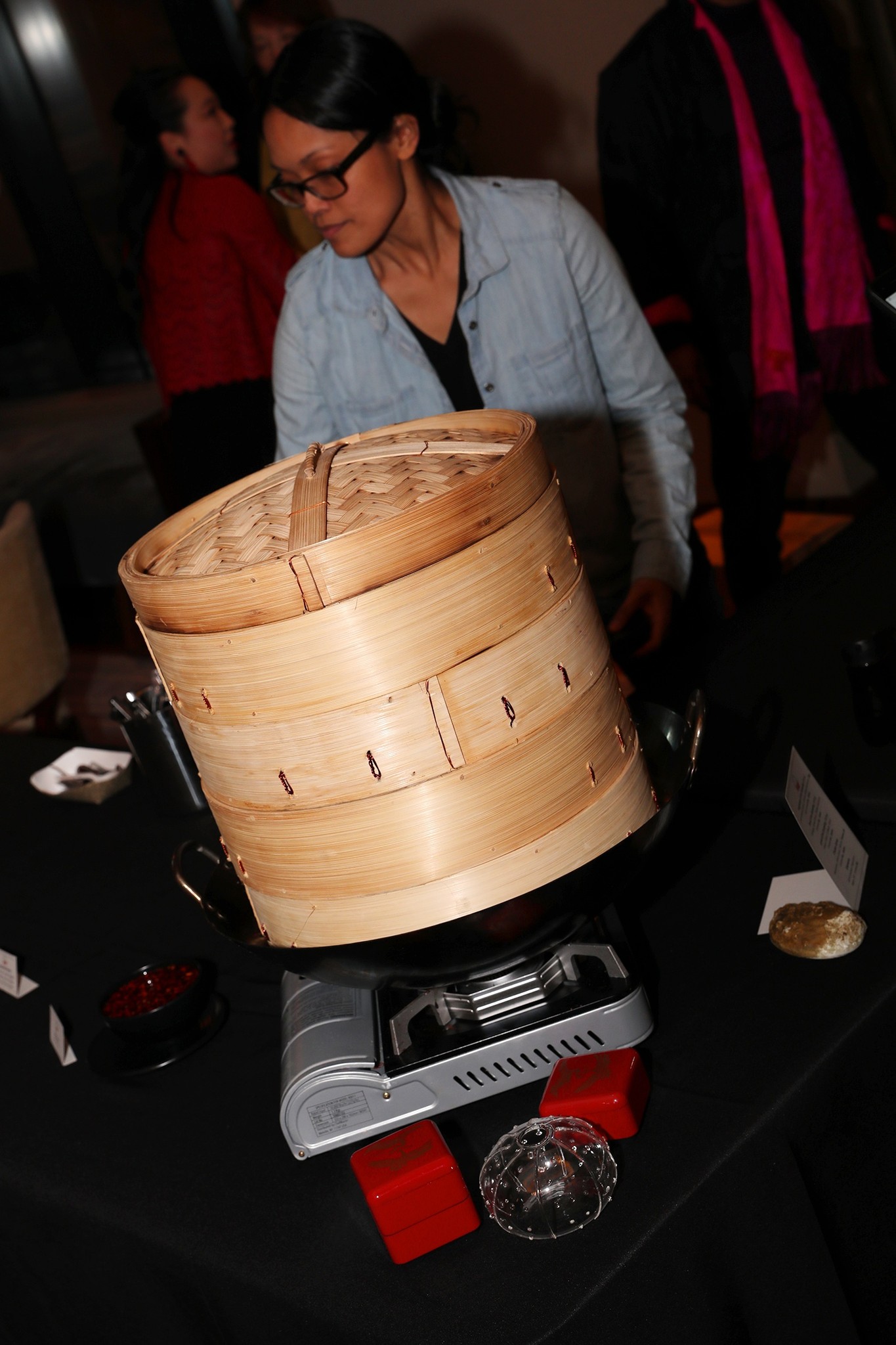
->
[255,15,732,718]
[587,0,892,608]
[127,62,299,504]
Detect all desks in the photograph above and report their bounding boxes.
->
[1,598,896,1344]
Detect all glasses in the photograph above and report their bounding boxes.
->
[267,109,394,209]
